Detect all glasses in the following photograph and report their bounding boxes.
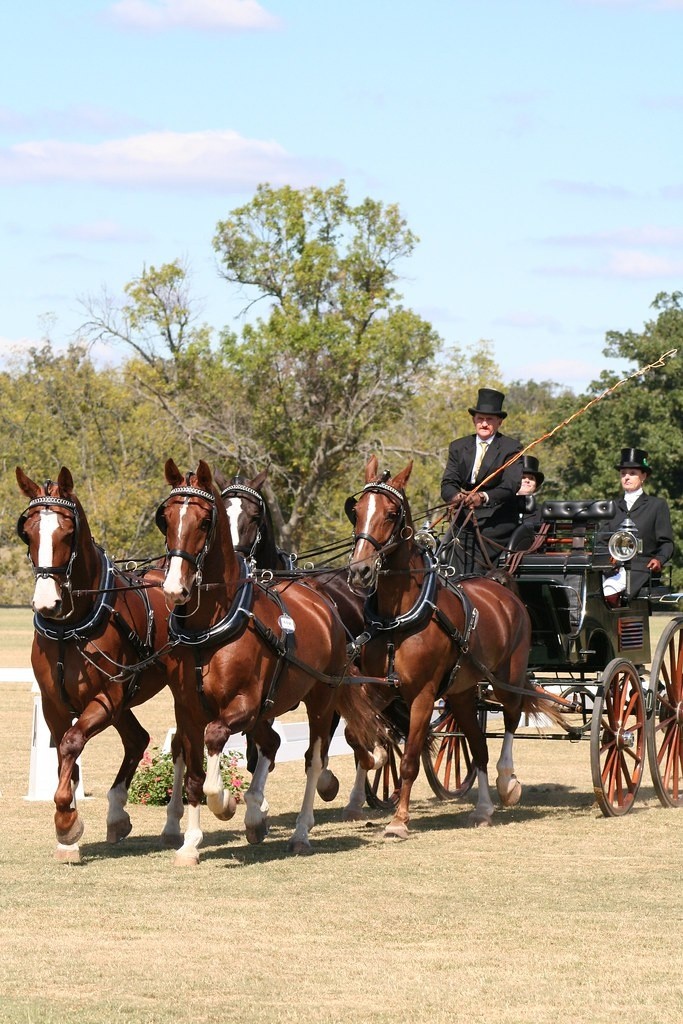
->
[522,475,536,482]
[621,470,641,477]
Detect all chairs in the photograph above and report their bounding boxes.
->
[498,496,615,571]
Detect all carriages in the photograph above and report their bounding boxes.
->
[13,455,683,866]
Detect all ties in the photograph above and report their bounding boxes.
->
[473,442,489,482]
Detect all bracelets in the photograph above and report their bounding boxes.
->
[478,492,485,508]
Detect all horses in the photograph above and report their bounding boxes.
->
[156,459,396,868]
[156,460,341,841]
[337,453,533,842]
[13,465,191,865]
[212,468,389,823]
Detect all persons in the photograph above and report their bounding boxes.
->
[517,455,544,552]
[440,387,525,581]
[602,448,674,609]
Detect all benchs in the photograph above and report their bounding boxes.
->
[535,523,672,601]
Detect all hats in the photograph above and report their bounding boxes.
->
[614,447,654,477]
[468,388,507,419]
[520,456,544,488]
